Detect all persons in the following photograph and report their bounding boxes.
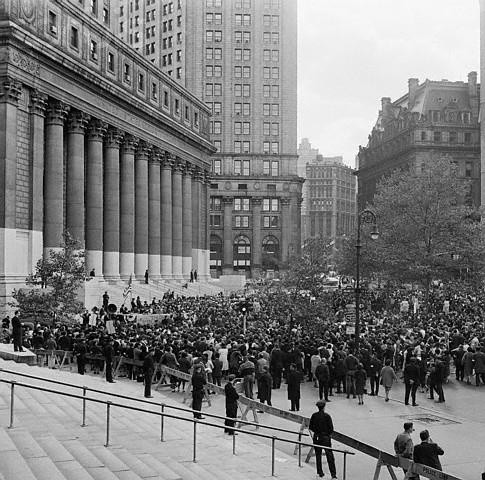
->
[142,349,155,397]
[189,268,193,283]
[412,429,445,472]
[190,365,205,418]
[0,282,484,406]
[194,269,198,282]
[144,269,149,284]
[89,268,96,277]
[393,421,422,480]
[224,374,239,435]
[307,399,339,480]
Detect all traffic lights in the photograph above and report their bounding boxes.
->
[456,255,460,261]
[452,255,455,261]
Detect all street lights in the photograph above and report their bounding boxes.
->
[239,305,248,334]
[354,210,381,355]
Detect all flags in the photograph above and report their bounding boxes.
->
[122,274,132,299]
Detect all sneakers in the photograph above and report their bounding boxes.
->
[331,474,338,480]
[224,430,239,436]
[19,350,27,352]
[144,394,154,398]
[14,349,19,352]
[318,386,453,407]
[242,386,301,414]
[193,416,206,420]
[319,473,324,477]
[106,379,117,383]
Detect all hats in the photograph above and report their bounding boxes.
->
[228,374,236,382]
[193,363,204,370]
[315,399,327,409]
